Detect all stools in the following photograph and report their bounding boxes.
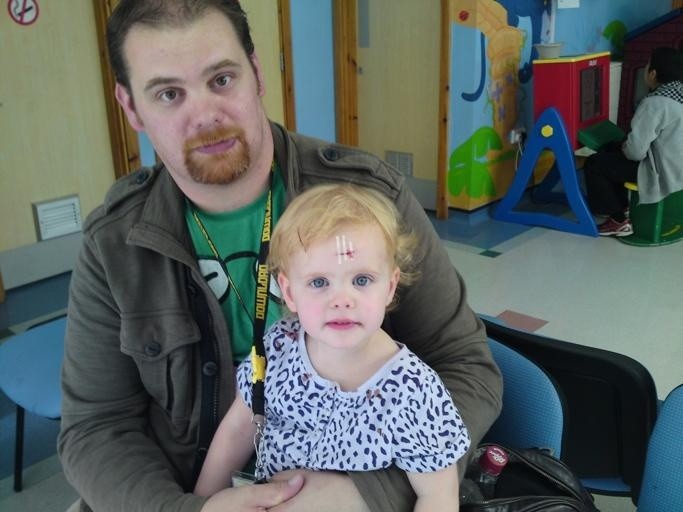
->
[2,313,72,496]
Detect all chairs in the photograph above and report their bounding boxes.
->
[470,309,683,512]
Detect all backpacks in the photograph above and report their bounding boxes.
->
[461,443,600,512]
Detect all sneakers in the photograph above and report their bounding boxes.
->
[593,201,634,236]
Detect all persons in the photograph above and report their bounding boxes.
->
[584,47,683,237]
[57,0,504,512]
[191,182,471,512]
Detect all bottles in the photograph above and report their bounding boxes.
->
[469,445,508,501]
